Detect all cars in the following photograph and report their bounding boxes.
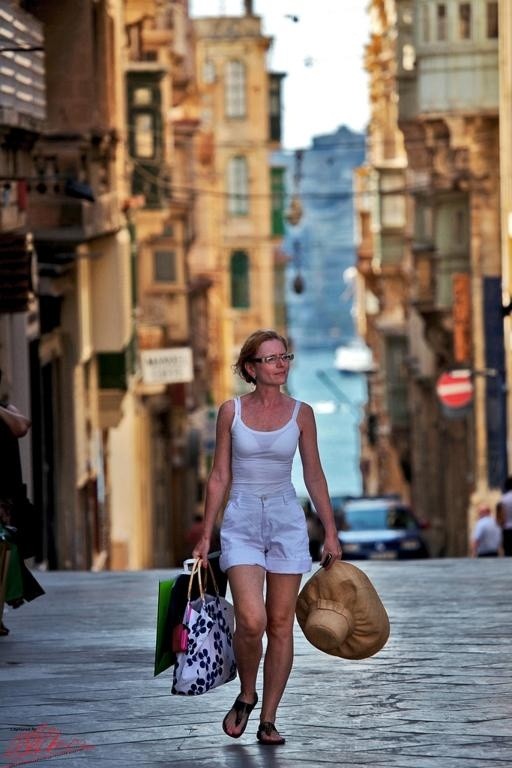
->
[333,497,434,559]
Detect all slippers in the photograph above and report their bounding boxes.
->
[257,721,285,744]
[223,691,258,737]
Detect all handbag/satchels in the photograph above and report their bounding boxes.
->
[154,556,238,696]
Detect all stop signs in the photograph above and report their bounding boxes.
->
[431,367,477,410]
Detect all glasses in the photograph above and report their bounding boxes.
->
[252,352,294,364]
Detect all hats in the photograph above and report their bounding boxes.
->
[295,560,390,659]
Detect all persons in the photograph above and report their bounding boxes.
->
[192,330,342,744]
[496,476,512,556]
[387,492,430,529]
[185,514,204,544]
[472,505,503,556]
[0,390,46,635]
